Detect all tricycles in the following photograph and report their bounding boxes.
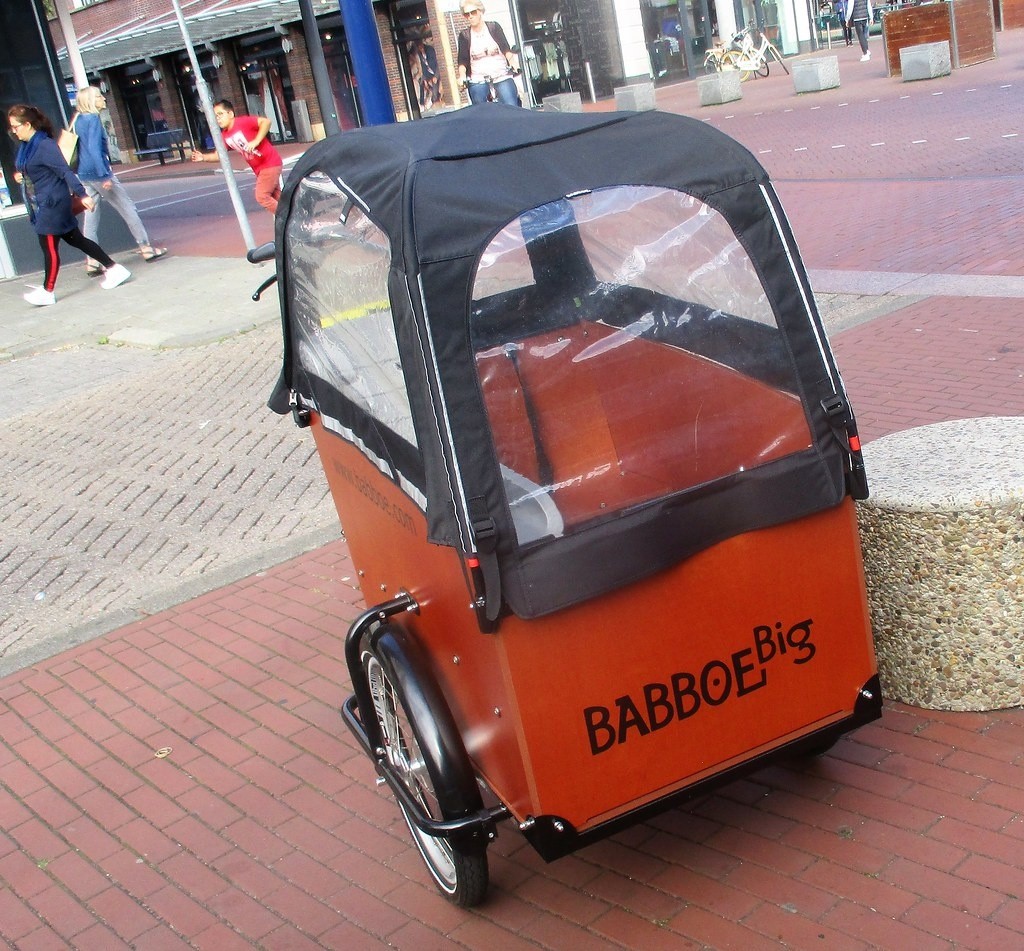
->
[244,101,886,902]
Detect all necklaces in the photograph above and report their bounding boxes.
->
[28,132,37,140]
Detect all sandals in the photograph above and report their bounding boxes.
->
[142,247,168,260]
[85,262,107,277]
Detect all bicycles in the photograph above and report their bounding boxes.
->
[703,17,790,84]
[459,45,520,104]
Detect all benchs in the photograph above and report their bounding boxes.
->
[134,129,190,166]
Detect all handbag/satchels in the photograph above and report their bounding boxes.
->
[847,20,854,28]
[67,194,87,214]
[55,128,79,169]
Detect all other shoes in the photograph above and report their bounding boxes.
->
[23,286,55,305]
[101,263,131,290]
[862,50,871,57]
[860,56,870,61]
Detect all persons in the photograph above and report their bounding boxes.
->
[192,101,284,214]
[10,104,131,306]
[456,0,519,107]
[76,86,168,277]
[821,0,873,61]
[408,35,440,112]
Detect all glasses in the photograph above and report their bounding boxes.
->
[463,9,480,19]
